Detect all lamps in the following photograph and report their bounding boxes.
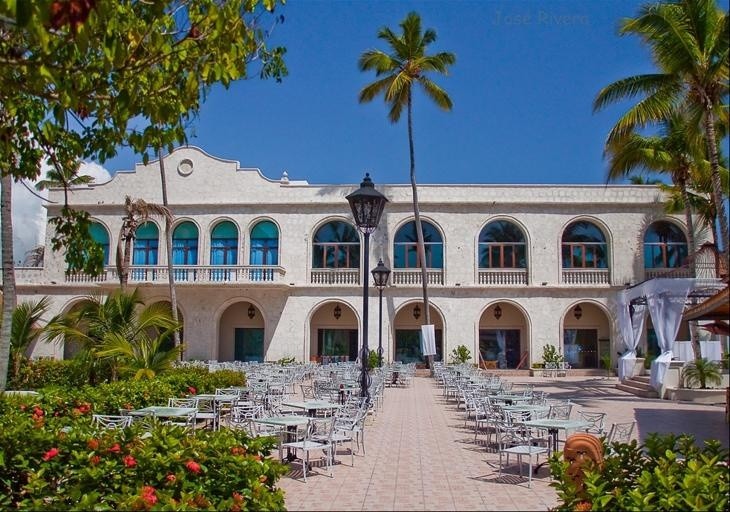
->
[413,304,421,320]
[334,302,341,319]
[247,304,255,319]
[494,304,501,320]
[574,304,582,320]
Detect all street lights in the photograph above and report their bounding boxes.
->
[347,172,389,408]
[372,256,392,368]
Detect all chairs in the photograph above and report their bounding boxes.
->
[433,362,635,489]
[90,358,415,484]
